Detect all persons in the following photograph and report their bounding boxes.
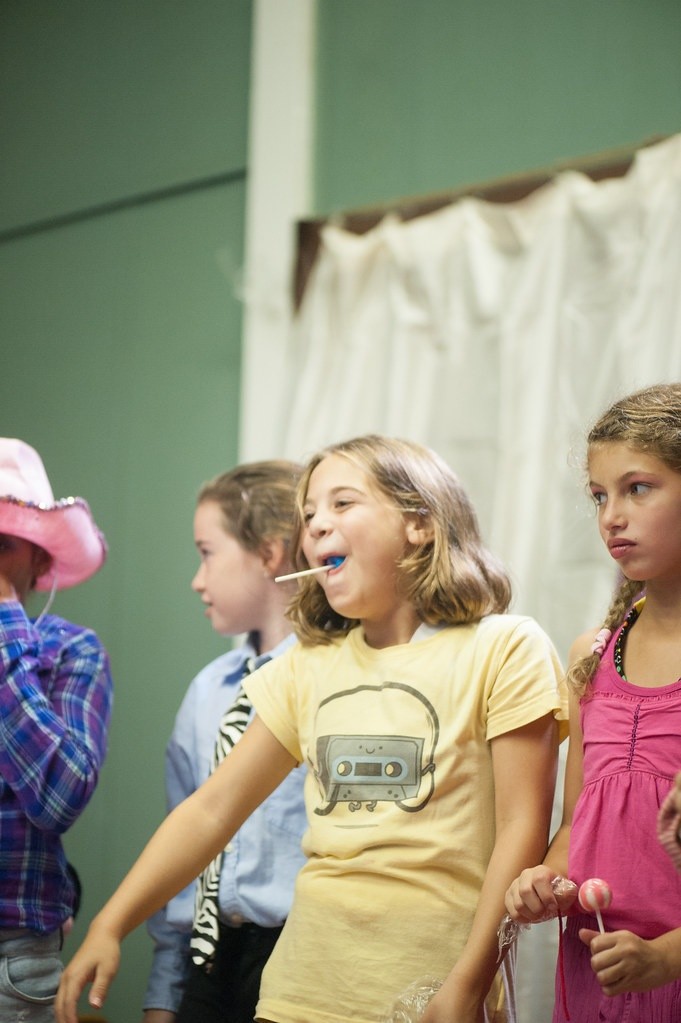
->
[504,382,681,1023]
[54,432,571,1022]
[141,459,325,1023]
[0,433,117,1023]
[657,766,681,882]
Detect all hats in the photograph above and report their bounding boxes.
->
[0,434,109,595]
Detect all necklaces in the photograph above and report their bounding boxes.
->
[618,594,648,694]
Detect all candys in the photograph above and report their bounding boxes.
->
[579,878,612,934]
[275,557,345,582]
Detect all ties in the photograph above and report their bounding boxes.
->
[188,661,261,969]
